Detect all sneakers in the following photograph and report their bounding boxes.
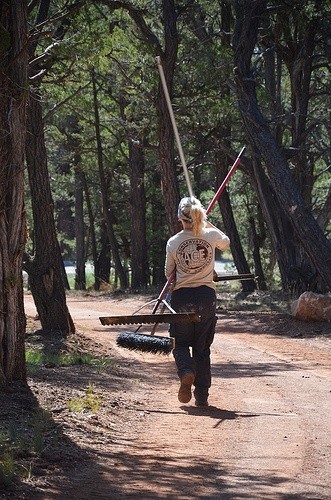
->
[193,386,209,406]
[177,366,195,403]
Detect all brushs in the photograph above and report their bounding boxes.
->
[115,331,175,357]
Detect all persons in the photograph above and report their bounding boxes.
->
[164,197,231,407]
